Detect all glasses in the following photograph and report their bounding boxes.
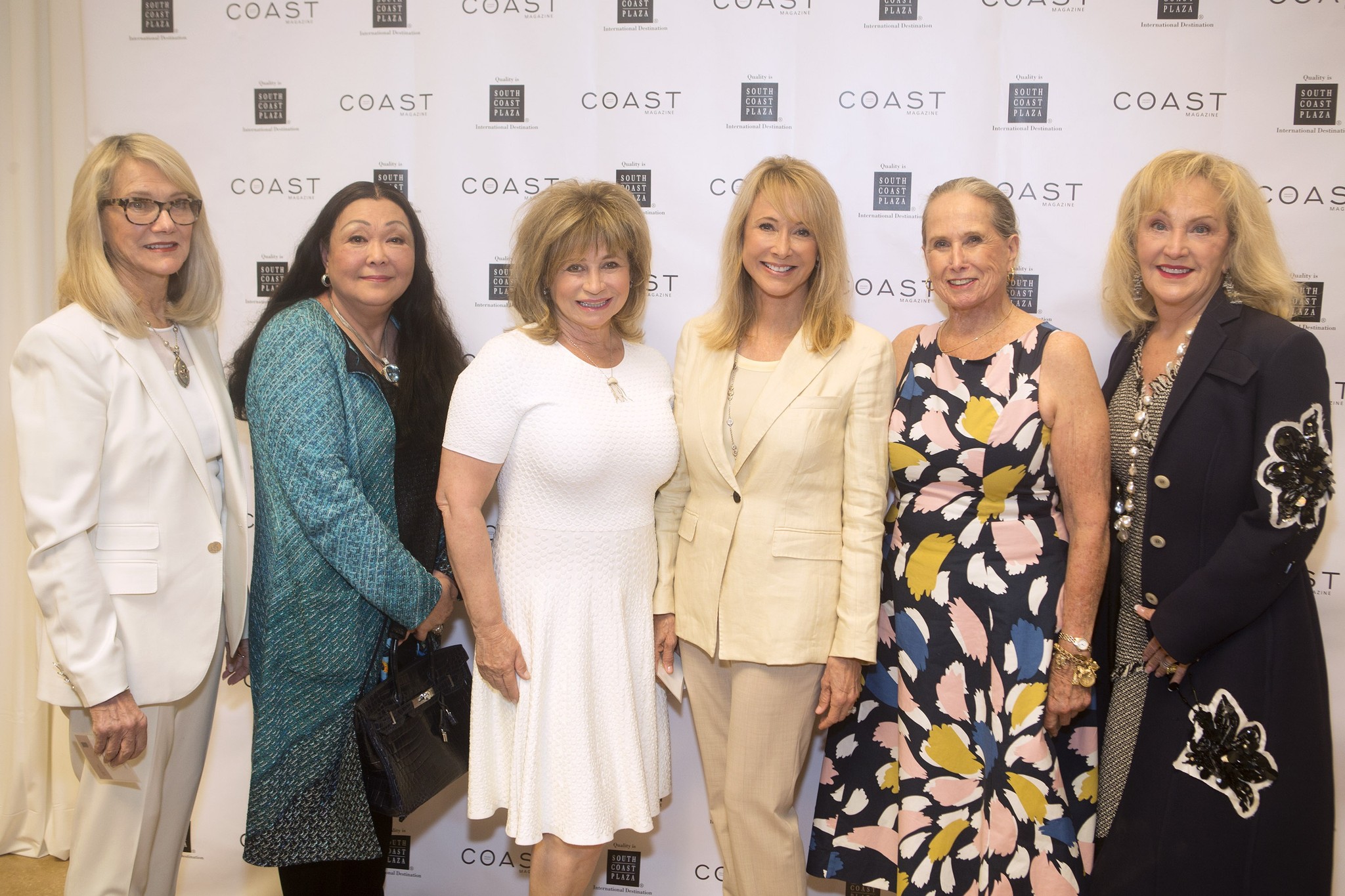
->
[98,197,203,225]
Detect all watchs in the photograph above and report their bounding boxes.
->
[1058,632,1093,653]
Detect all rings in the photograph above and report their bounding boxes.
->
[432,625,443,635]
[1160,658,1179,674]
[848,706,857,715]
[236,652,245,659]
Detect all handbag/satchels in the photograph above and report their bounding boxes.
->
[355,623,473,818]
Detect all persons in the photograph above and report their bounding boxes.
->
[435,183,680,896]
[11,135,255,896]
[654,157,897,896]
[229,182,470,896]
[807,180,1109,896]
[1098,152,1334,896]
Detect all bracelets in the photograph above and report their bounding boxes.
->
[1054,643,1099,687]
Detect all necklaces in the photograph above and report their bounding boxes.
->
[558,324,633,404]
[939,303,1014,353]
[727,337,742,460]
[326,286,400,383]
[1115,316,1200,541]
[145,321,190,388]
